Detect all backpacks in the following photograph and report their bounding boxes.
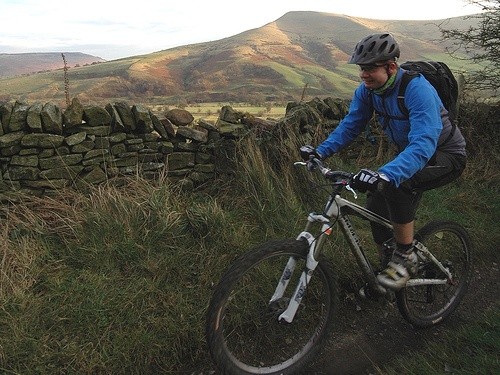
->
[397,59,460,131]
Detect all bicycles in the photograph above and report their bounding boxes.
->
[205,152,476,375]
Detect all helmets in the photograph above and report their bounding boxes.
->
[349,30,402,64]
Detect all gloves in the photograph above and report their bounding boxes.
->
[299,144,322,171]
[350,165,389,194]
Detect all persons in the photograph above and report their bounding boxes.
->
[298,33,466,300]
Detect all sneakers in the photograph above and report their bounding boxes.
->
[359,281,389,298]
[375,248,421,288]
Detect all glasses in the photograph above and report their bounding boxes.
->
[359,63,387,73]
